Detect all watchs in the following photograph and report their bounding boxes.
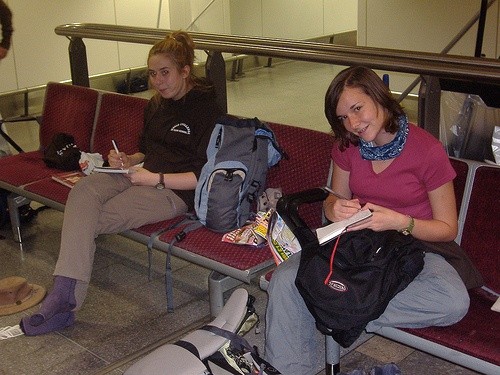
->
[399,215,414,237]
[155,172,167,189]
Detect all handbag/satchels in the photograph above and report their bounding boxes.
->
[295,228,428,348]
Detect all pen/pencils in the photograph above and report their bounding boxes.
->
[111,139,123,166]
[324,186,350,201]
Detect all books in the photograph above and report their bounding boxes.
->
[95,161,144,174]
[316,209,373,245]
[52,170,88,188]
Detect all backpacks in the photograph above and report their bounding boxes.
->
[193,115,286,233]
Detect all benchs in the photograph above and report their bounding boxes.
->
[0,79,500,375]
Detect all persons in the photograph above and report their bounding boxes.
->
[19,30,224,336]
[0,0,14,60]
[265,66,470,375]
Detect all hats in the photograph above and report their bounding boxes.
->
[0,276,47,316]
